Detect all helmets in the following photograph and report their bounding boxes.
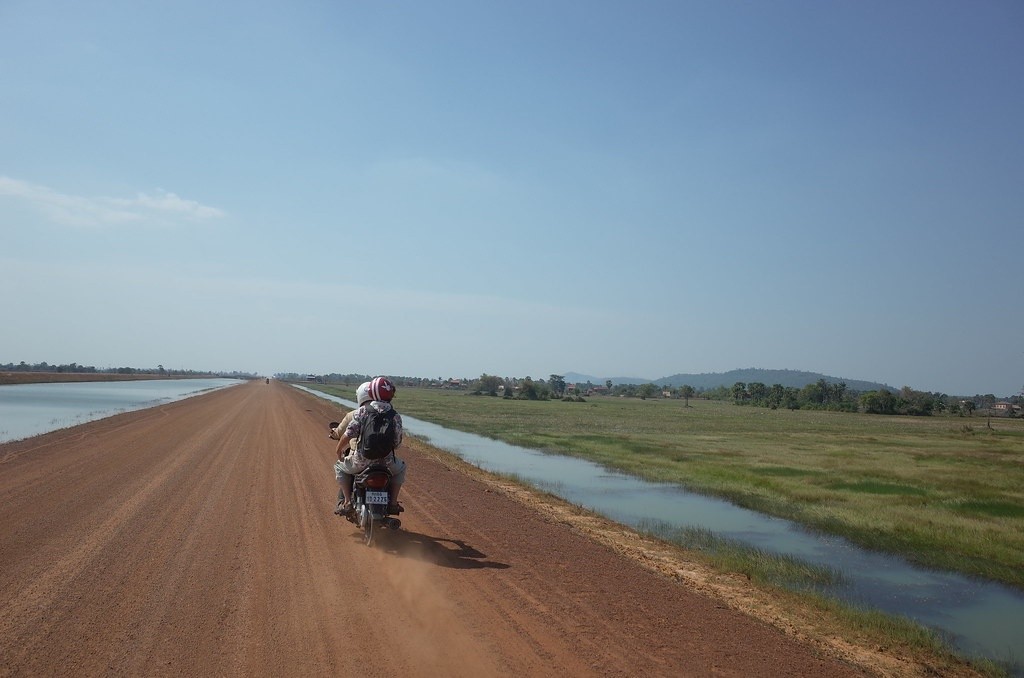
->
[368,377,395,403]
[355,382,373,407]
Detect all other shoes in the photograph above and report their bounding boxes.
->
[387,504,404,514]
[334,502,354,515]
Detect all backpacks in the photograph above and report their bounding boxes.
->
[357,402,396,460]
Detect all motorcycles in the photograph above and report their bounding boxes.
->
[328,421,405,548]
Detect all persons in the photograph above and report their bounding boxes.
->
[329,382,374,509]
[333,377,407,516]
